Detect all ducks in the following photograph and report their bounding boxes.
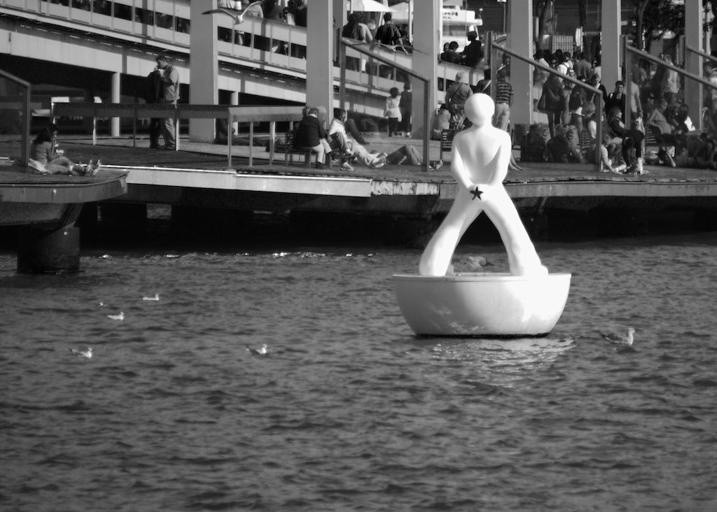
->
[600,326,638,346]
[142,293,160,301]
[245,344,269,357]
[107,312,126,321]
[68,346,96,359]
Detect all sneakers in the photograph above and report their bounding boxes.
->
[435,159,444,169]
[149,141,176,150]
[314,150,355,173]
[85,158,101,177]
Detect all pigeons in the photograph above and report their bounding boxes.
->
[342,159,353,171]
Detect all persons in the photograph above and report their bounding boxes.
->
[52,126,101,177]
[145,68,164,149]
[31,122,93,176]
[156,55,179,150]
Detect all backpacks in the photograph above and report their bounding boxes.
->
[569,88,581,106]
[582,99,595,114]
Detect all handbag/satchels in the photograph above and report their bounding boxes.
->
[537,92,548,113]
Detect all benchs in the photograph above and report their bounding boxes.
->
[579,129,686,167]
[440,130,456,160]
[267,139,357,167]
